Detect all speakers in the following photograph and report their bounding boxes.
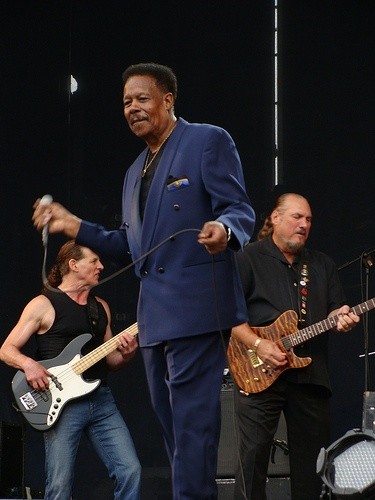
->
[216,391,291,477]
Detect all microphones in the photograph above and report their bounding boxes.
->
[39,194,53,246]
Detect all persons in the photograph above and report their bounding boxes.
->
[231,193,360,500]
[31,62,256,500]
[0,239,142,500]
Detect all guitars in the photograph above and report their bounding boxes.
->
[226,297,375,394]
[11,319,139,432]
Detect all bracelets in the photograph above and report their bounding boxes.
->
[252,339,260,354]
[225,226,231,241]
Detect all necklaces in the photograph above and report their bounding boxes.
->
[142,124,174,178]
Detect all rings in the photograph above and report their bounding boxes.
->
[344,325,349,329]
